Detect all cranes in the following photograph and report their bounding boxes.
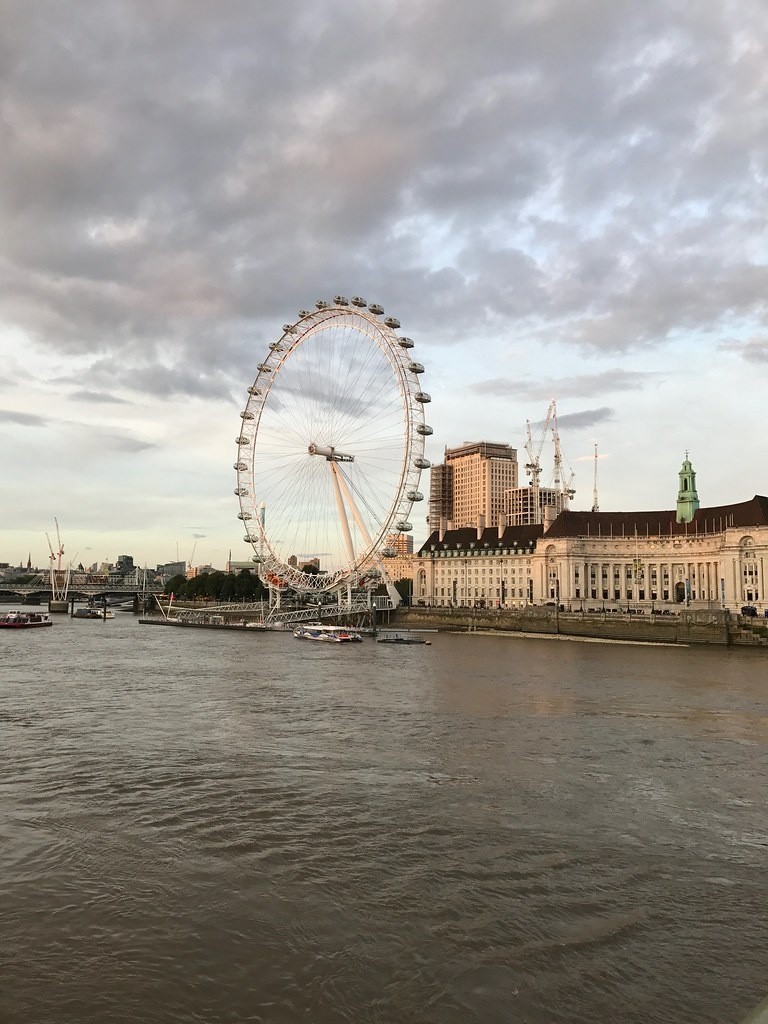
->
[524,399,600,526]
[44,517,66,586]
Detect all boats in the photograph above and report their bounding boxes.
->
[0,610,53,627]
[293,630,364,645]
